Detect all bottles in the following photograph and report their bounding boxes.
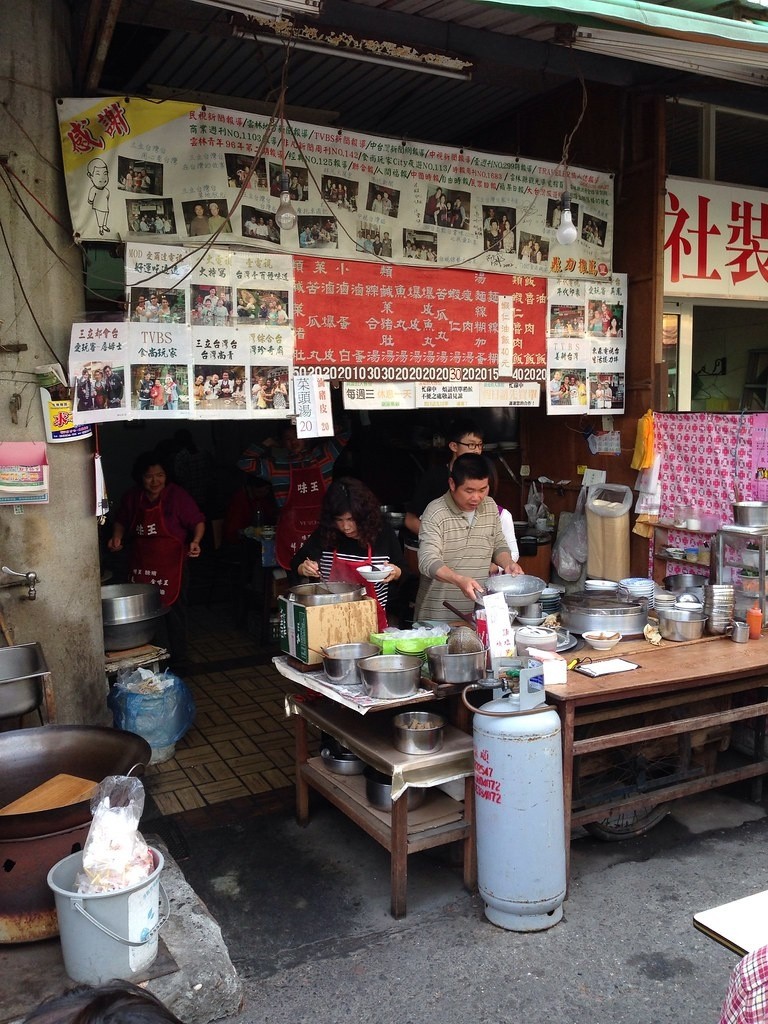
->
[673,502,719,535]
[746,601,763,640]
[685,547,711,566]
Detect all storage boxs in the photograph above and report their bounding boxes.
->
[277,594,378,665]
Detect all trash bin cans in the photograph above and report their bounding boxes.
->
[114,675,181,760]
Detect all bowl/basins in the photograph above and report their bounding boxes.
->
[357,656,424,698]
[581,574,735,650]
[356,564,394,582]
[424,643,490,683]
[730,500,768,528]
[362,764,428,812]
[739,545,768,598]
[393,711,448,755]
[396,640,428,664]
[484,573,563,656]
[483,444,497,448]
[319,642,381,684]
[320,748,368,775]
[501,442,516,449]
[665,547,686,558]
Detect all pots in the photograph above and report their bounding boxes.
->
[0,721,157,839]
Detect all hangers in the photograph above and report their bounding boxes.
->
[703,374,729,400]
[692,386,711,400]
[696,365,711,377]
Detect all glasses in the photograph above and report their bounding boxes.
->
[453,440,485,450]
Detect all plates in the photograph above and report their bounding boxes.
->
[513,521,530,535]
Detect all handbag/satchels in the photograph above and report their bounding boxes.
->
[550,483,588,582]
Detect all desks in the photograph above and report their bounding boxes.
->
[420,619,768,900]
[105,644,170,695]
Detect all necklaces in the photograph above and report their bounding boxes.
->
[371,191,391,216]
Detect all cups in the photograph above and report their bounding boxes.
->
[725,621,750,643]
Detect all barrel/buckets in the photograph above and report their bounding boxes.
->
[47,844,171,988]
[288,581,364,607]
[514,529,553,588]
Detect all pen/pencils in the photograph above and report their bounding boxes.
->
[567,658,580,670]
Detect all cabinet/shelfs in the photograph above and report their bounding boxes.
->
[272,654,485,920]
[716,528,768,629]
[647,521,719,585]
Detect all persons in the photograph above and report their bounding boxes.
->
[414,453,524,622]
[482,456,520,575]
[289,477,406,627]
[484,208,514,254]
[125,169,151,189]
[324,179,347,203]
[551,302,621,409]
[232,166,258,190]
[583,220,599,245]
[520,238,542,264]
[414,418,499,500]
[405,239,436,262]
[128,202,392,257]
[552,201,562,229]
[236,420,351,584]
[77,288,288,412]
[271,169,302,201]
[107,451,206,679]
[425,187,466,229]
